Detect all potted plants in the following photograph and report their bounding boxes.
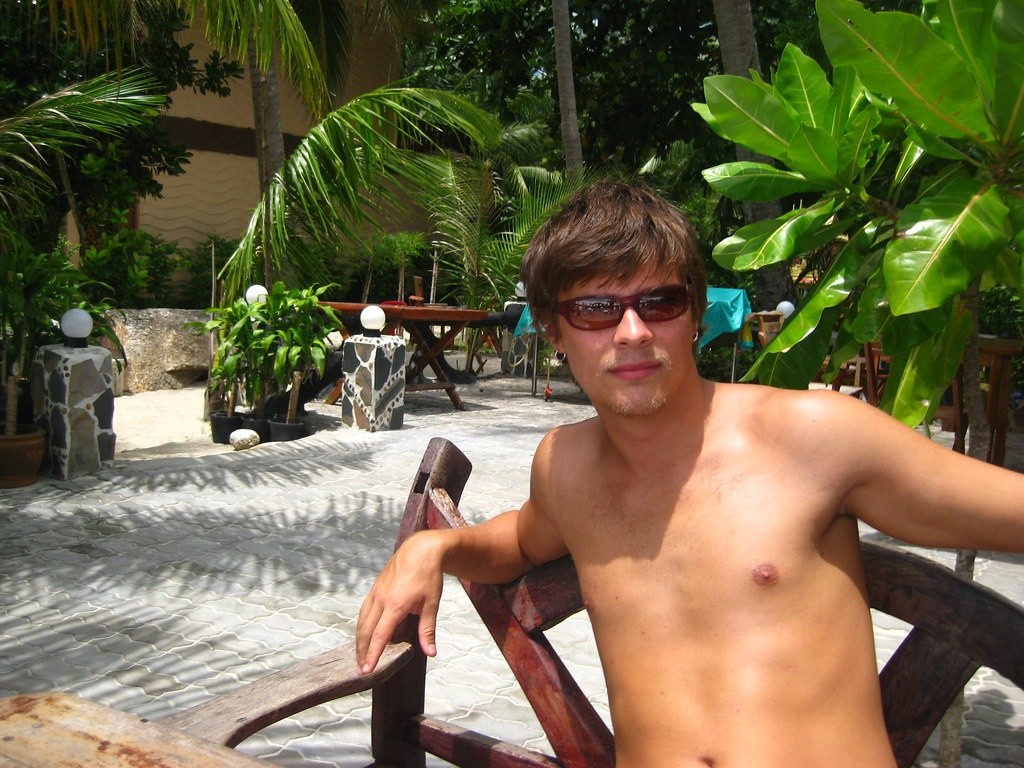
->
[182,280,346,446]
[0,222,89,493]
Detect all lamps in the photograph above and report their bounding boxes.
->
[59,307,94,349]
[359,306,388,337]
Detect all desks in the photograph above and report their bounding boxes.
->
[312,299,489,413]
[514,289,750,404]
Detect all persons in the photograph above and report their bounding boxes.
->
[356,176,1024,768]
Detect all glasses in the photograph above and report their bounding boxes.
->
[553,284,692,330]
